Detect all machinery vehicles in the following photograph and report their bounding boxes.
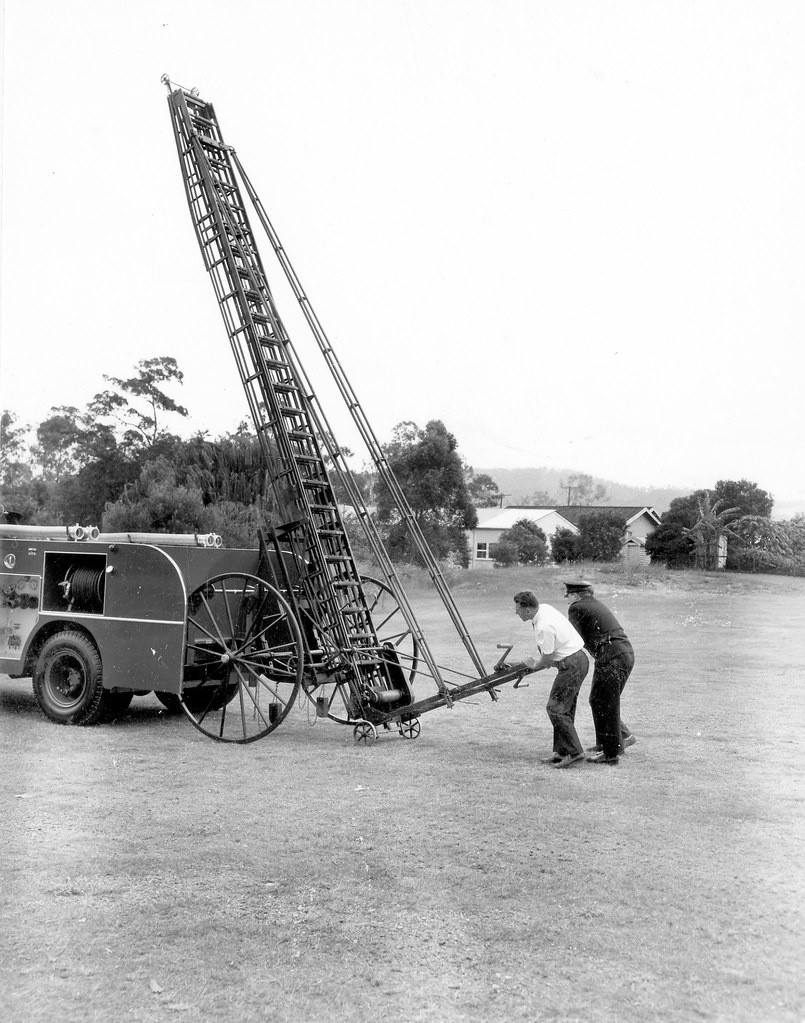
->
[0,511,330,727]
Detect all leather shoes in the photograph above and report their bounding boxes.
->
[586,753,620,765]
[587,746,602,753]
[622,735,637,749]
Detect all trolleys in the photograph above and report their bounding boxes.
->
[299,626,548,748]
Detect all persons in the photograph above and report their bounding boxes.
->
[514,592,589,768]
[564,580,635,764]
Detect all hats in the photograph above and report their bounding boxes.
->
[564,580,592,598]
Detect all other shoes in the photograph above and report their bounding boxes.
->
[555,752,584,769]
[542,753,563,765]
